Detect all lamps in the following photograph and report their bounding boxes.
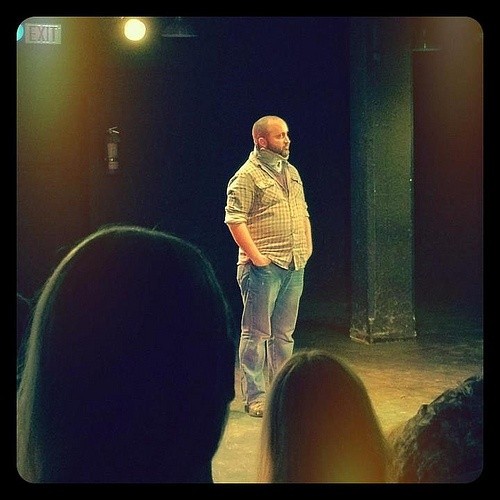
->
[122,18,149,46]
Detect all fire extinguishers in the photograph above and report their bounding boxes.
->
[103,125,123,176]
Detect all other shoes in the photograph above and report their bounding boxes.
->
[244,401,266,417]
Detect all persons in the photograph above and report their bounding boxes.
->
[259,346,393,483]
[223,116,314,418]
[387,377,485,482]
[17,222,238,484]
